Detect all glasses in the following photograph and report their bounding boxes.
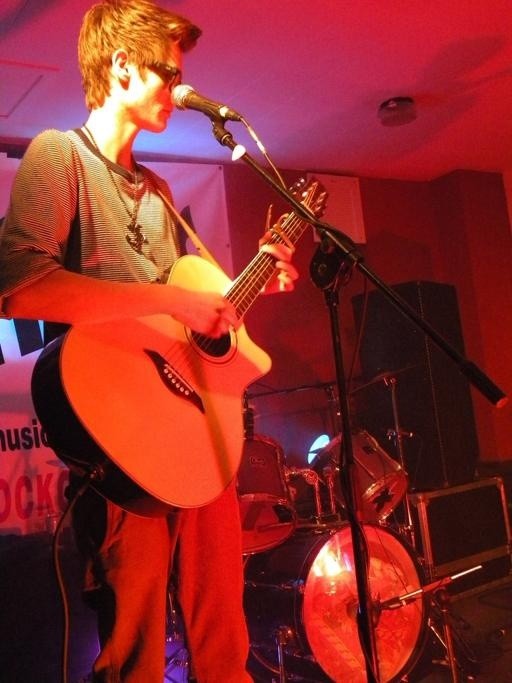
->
[145,56,184,91]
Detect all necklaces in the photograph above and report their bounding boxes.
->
[85,123,147,251]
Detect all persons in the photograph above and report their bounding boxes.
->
[0,0,299,683]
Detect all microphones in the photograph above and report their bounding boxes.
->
[171,84,243,121]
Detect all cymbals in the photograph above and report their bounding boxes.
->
[351,365,421,391]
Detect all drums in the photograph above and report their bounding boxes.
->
[305,427,409,520]
[235,434,301,556]
[244,520,429,677]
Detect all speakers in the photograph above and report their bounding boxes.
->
[352,280,479,491]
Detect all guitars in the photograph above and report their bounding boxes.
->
[30,175,329,516]
